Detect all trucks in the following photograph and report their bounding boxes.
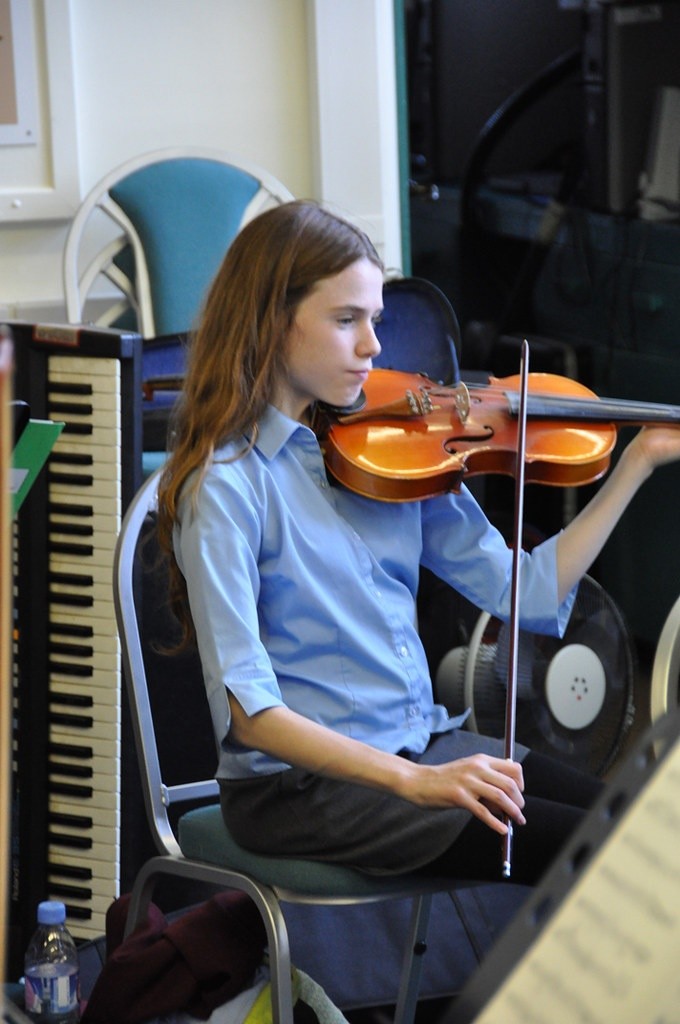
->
[455,23,680,407]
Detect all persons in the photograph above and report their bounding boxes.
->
[148,197,680,888]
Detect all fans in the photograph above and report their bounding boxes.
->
[432,569,639,781]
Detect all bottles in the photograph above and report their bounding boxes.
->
[24,900,80,1024]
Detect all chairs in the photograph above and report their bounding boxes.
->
[59,139,295,352]
[108,452,494,1024]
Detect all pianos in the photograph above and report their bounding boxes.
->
[11,318,142,952]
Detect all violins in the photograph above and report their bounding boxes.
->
[312,366,680,504]
